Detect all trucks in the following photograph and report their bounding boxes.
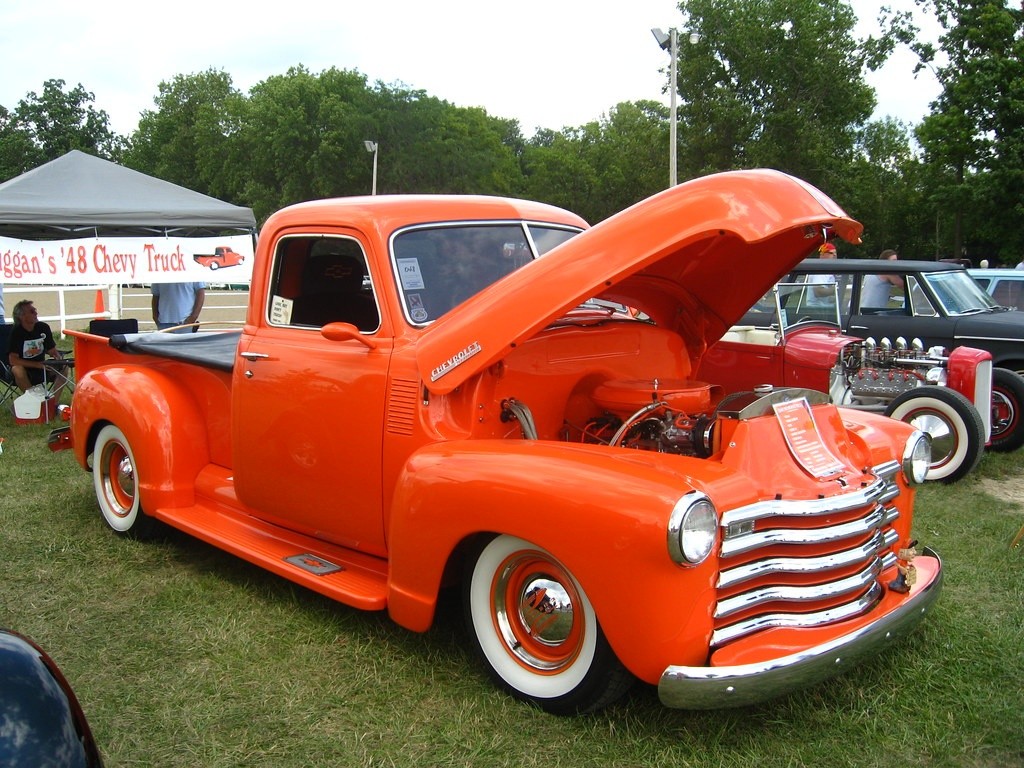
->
[193,245,244,270]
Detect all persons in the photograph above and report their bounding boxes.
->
[807,242,838,306]
[860,249,905,308]
[9,300,69,413]
[0,282,5,324]
[151,282,206,333]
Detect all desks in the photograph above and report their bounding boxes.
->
[41,359,75,425]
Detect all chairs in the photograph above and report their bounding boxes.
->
[89,318,139,338]
[293,254,363,329]
[0,323,22,416]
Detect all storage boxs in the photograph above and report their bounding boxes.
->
[16,395,56,425]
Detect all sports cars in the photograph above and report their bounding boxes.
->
[691,280,1022,488]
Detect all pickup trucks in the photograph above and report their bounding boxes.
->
[42,168,947,717]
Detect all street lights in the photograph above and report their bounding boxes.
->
[650,26,703,189]
[362,141,378,196]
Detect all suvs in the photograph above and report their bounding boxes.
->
[729,256,1024,385]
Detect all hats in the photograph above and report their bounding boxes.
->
[879,250,896,259]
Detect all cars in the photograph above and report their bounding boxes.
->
[901,268,1024,316]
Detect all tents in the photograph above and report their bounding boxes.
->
[0,150,258,338]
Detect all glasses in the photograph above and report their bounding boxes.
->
[23,308,37,313]
[824,251,837,255]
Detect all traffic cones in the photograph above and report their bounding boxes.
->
[93,290,105,321]
